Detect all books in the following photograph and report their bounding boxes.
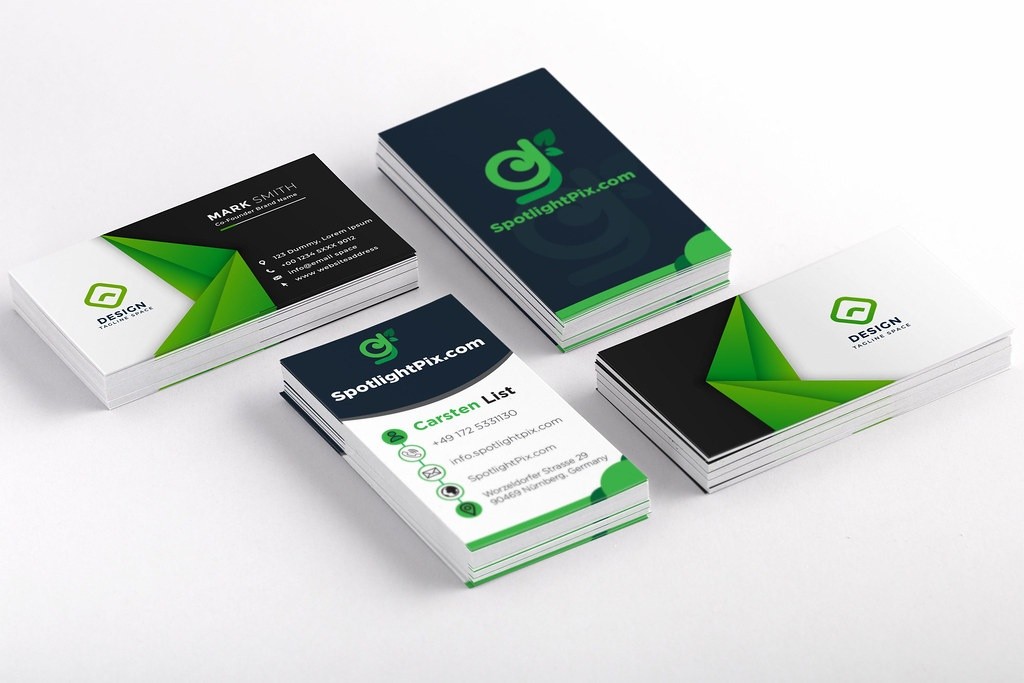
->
[8,154,420,412]
[376,67,734,351]
[278,294,653,589]
[596,225,1016,492]
